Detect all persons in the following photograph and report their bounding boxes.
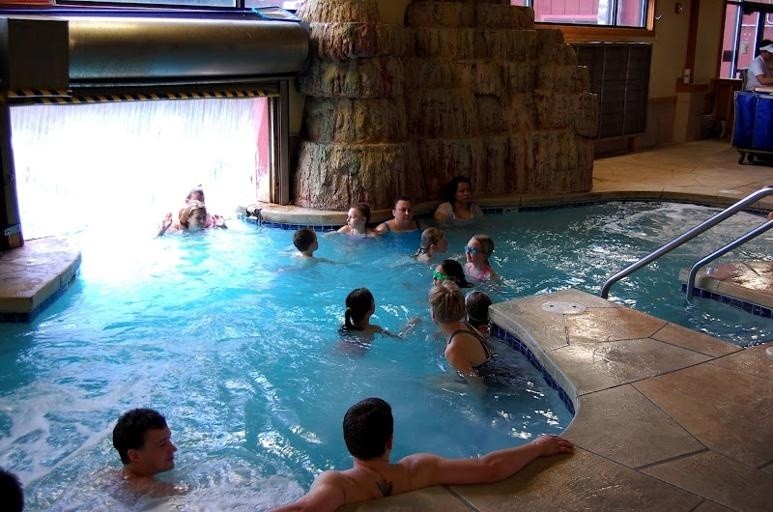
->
[373,196,432,235]
[155,199,229,239]
[268,396,575,512]
[95,407,193,497]
[745,39,772,92]
[334,287,421,355]
[175,188,213,222]
[292,227,333,265]
[435,173,486,223]
[1,467,24,512]
[410,226,524,398]
[325,201,376,240]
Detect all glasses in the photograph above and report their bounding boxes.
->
[432,270,449,280]
[464,245,483,254]
